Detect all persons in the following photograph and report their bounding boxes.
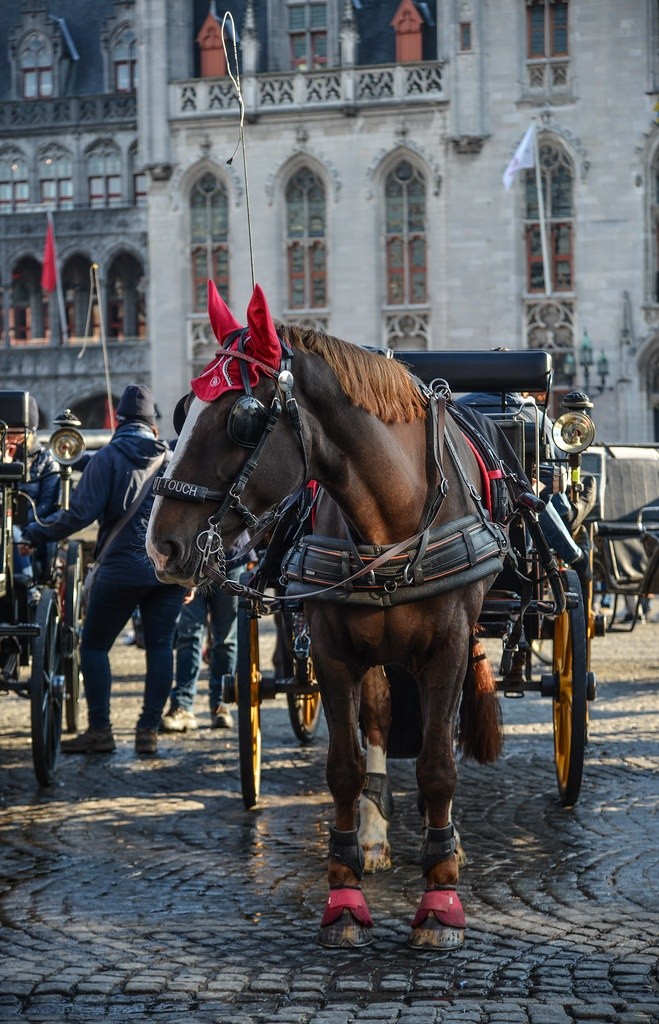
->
[0,386,257,754]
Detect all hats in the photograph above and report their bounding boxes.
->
[28,395,40,433]
[115,384,157,426]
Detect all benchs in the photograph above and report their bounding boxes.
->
[393,350,552,636]
[0,390,29,483]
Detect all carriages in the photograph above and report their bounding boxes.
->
[144,278,597,959]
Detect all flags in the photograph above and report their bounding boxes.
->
[42,222,57,291]
[502,127,535,190]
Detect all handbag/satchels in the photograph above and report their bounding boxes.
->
[82,564,102,607]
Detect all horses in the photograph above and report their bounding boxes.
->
[145,281,511,948]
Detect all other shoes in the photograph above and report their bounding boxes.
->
[135,625,146,649]
[210,705,234,728]
[135,727,158,754]
[60,726,116,753]
[161,706,198,731]
[568,475,597,533]
